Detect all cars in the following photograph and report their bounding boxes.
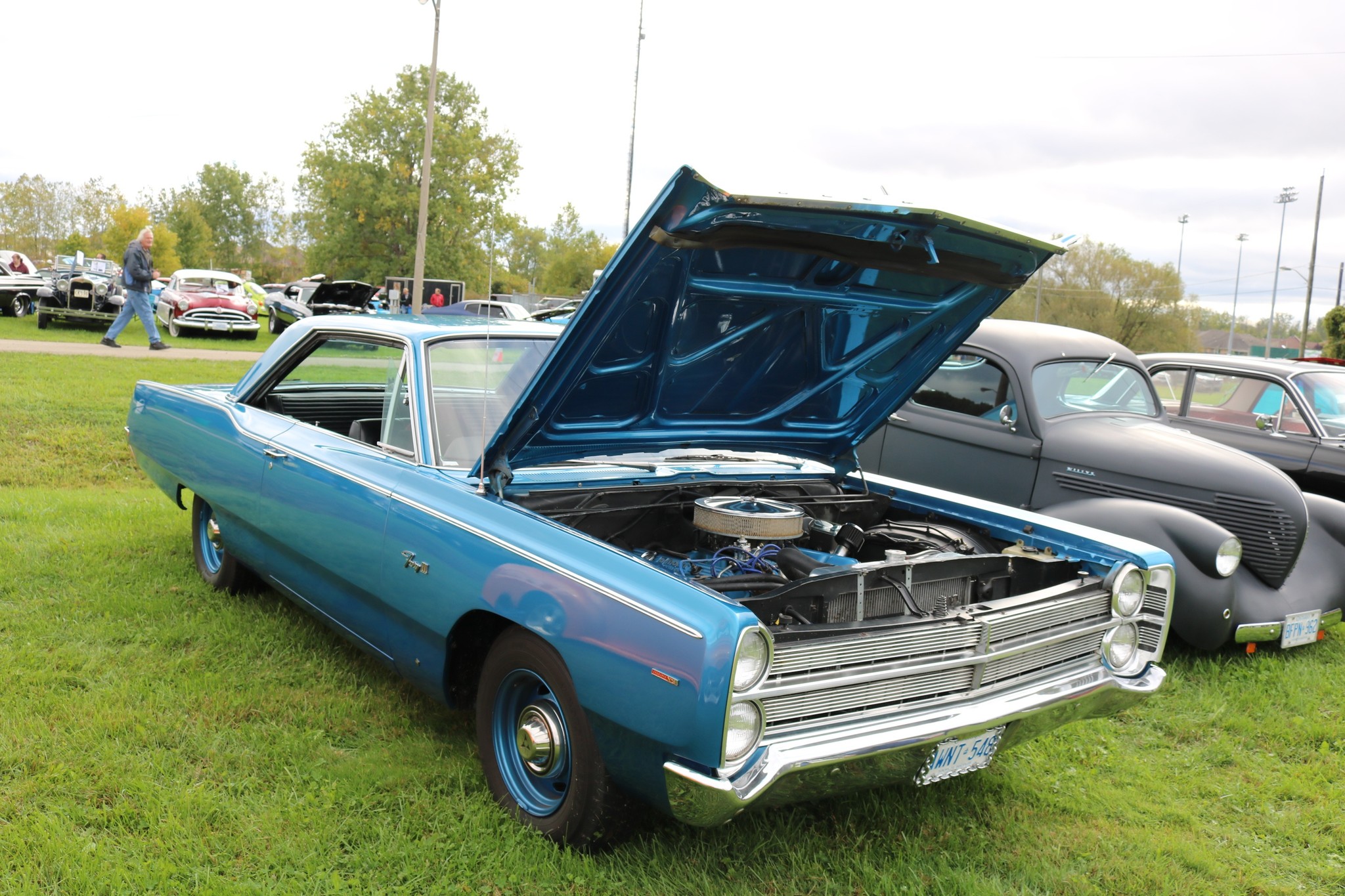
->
[213,274,585,351]
[856,316,1345,661]
[0,250,261,341]
[124,164,1178,853]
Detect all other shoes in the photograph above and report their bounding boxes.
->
[100,337,121,347]
[149,341,171,350]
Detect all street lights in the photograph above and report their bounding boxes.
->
[1265,186,1299,357]
[1228,233,1249,353]
[1278,265,1314,357]
[1178,213,1190,273]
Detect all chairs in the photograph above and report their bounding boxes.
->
[349,418,414,452]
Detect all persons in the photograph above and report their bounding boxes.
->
[99,227,173,350]
[8,254,30,275]
[379,288,444,314]
[96,253,106,260]
